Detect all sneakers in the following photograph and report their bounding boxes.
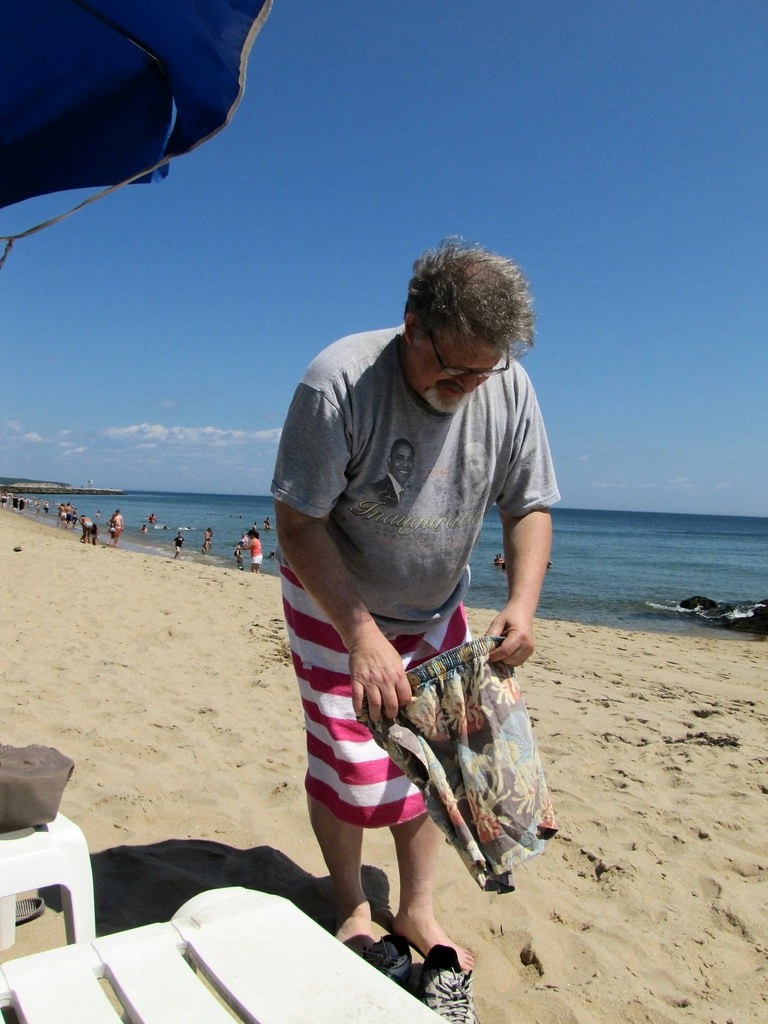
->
[419,944,479,1024]
[362,933,413,987]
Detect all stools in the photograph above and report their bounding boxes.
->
[0,808,97,946]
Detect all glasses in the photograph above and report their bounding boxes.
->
[413,314,510,379]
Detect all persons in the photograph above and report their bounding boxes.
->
[203,538,211,554]
[235,544,244,571]
[270,232,562,980]
[455,441,492,504]
[0,486,247,529]
[269,552,275,559]
[373,439,415,507]
[106,515,117,546]
[264,521,269,528]
[205,528,213,545]
[253,522,257,532]
[139,525,148,532]
[265,517,271,529]
[79,515,93,543]
[86,524,98,545]
[494,553,504,563]
[237,530,263,573]
[240,533,249,547]
[172,532,185,559]
[112,510,124,546]
